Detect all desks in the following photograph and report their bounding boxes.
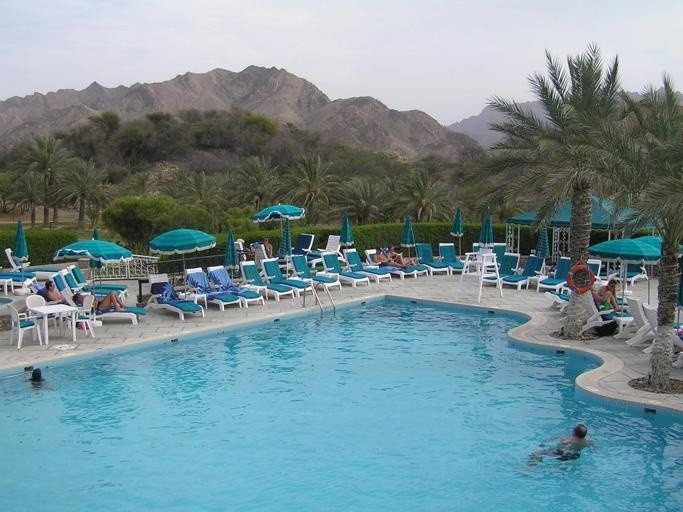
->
[364,265,379,270]
[289,276,314,290]
[30,304,80,345]
[245,284,267,300]
[178,291,208,313]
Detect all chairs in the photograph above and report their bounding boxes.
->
[145,274,205,320]
[240,261,295,302]
[2,268,37,285]
[1,278,14,294]
[57,269,124,305]
[258,257,313,297]
[185,268,241,311]
[390,239,683,369]
[321,252,370,288]
[207,266,265,307]
[27,284,147,324]
[63,294,97,339]
[343,249,390,283]
[7,304,42,349]
[2,275,33,289]
[364,250,417,280]
[290,256,342,292]
[26,295,58,339]
[234,233,343,268]
[53,275,115,306]
[5,248,61,280]
[70,264,130,299]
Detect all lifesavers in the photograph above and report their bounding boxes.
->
[568,264,594,293]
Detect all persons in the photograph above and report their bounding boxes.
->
[44,280,79,329]
[599,278,617,298]
[24,368,54,394]
[374,245,418,267]
[70,291,128,312]
[529,424,592,467]
[589,286,623,311]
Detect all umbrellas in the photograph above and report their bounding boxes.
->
[674,274,683,334]
[586,238,660,299]
[12,219,28,271]
[51,240,133,307]
[634,235,682,303]
[150,226,216,299]
[89,226,103,296]
[225,229,239,286]
